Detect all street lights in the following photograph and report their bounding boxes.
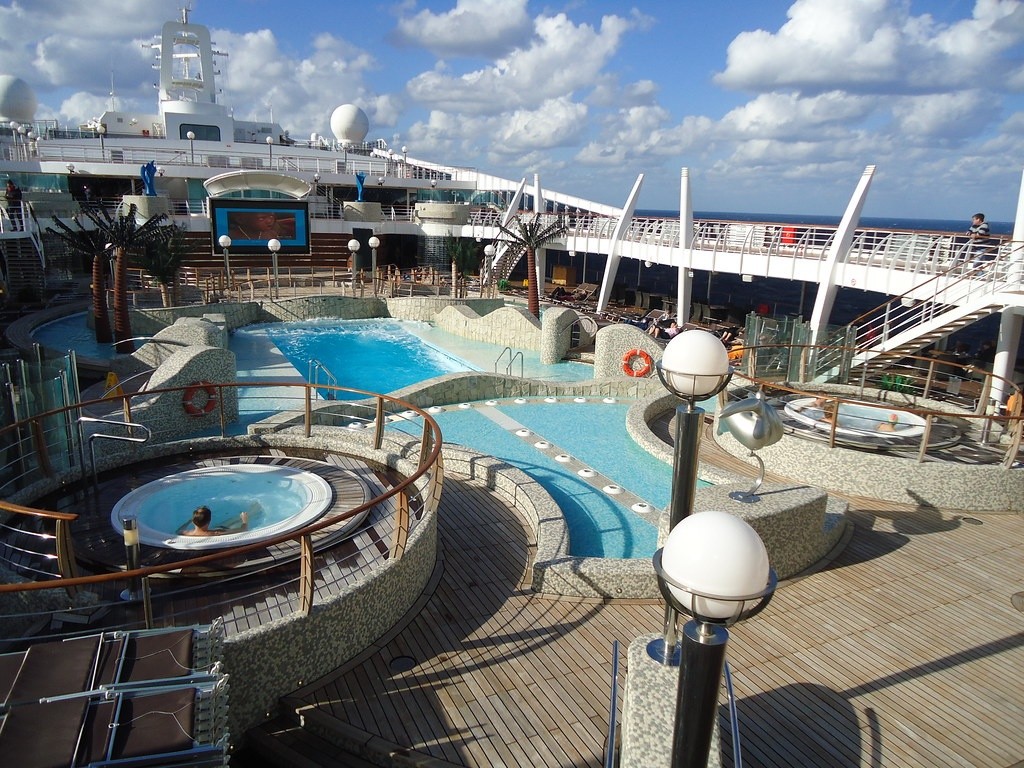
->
[267,238,281,299]
[388,149,395,170]
[647,329,734,667]
[97,126,105,160]
[10,121,44,161]
[105,243,115,285]
[368,236,380,297]
[347,239,360,296]
[266,136,273,170]
[652,510,777,768]
[484,245,496,299]
[401,146,408,163]
[187,131,195,166]
[342,142,348,174]
[219,235,231,292]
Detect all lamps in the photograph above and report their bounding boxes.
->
[569,250,578,257]
[644,261,652,268]
[157,167,166,178]
[66,162,75,175]
[377,177,385,187]
[742,275,754,283]
[430,180,437,188]
[314,172,321,183]
[476,237,483,242]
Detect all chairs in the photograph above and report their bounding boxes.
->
[543,280,780,350]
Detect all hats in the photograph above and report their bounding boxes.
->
[888,414,898,424]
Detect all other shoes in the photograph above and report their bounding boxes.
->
[10,228,17,231]
[20,228,24,231]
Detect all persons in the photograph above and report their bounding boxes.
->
[813,390,827,407]
[966,213,990,279]
[143,130,150,137]
[180,505,248,536]
[227,211,296,241]
[879,414,898,432]
[625,316,768,348]
[821,404,839,426]
[4,180,24,232]
[550,286,587,301]
[403,266,467,282]
[1005,395,1015,422]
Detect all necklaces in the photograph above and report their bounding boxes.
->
[238,226,261,240]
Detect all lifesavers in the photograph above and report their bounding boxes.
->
[623,348,652,378]
[183,380,217,418]
